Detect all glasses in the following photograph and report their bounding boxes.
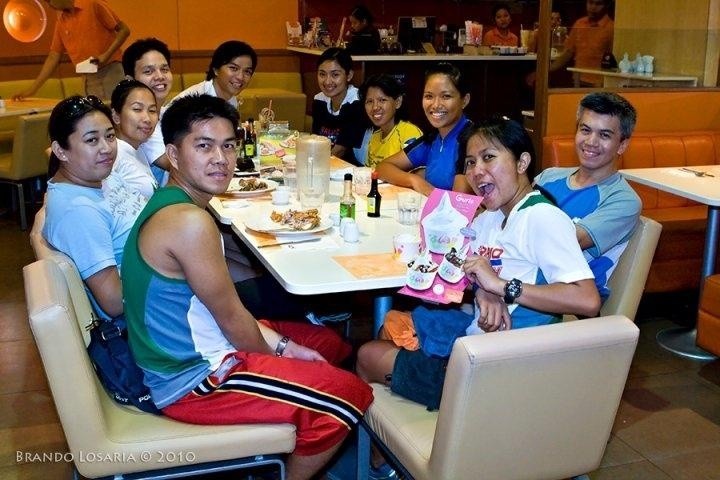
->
[62,94,103,124]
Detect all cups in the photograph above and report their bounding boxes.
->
[272,186,293,205]
[521,30,537,54]
[399,190,422,230]
[407,261,439,292]
[296,136,331,202]
[422,209,469,255]
[393,235,421,263]
[438,253,470,283]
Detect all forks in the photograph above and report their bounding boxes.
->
[678,168,712,176]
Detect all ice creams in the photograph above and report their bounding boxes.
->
[438,240,473,286]
[406,247,440,292]
[420,190,470,257]
[390,226,422,265]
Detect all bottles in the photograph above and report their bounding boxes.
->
[366,172,382,219]
[339,174,356,216]
[388,25,394,36]
[624,73,653,78]
[340,217,358,243]
[282,155,297,192]
[237,118,257,170]
[379,37,401,55]
[552,26,567,58]
[616,51,657,74]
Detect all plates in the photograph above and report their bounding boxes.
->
[279,137,295,149]
[248,207,335,235]
[220,178,279,198]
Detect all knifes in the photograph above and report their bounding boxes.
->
[682,167,715,178]
[254,235,324,248]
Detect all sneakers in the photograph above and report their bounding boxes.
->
[318,311,354,326]
[368,462,404,480]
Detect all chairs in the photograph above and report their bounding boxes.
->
[0,64,720,480]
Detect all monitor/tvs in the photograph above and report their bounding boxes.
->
[398,16,436,53]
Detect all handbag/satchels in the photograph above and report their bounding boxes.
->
[87,320,165,418]
